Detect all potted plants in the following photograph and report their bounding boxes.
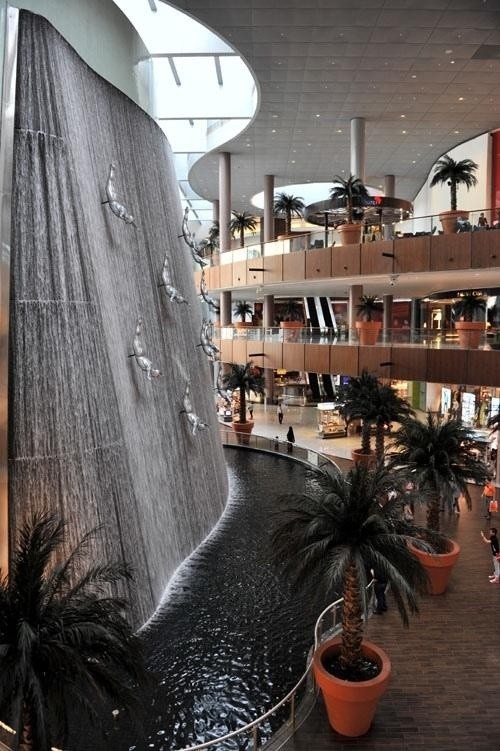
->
[351,294,385,346]
[326,175,375,245]
[428,154,479,233]
[232,298,257,341]
[219,359,267,445]
[339,371,491,596]
[271,456,438,737]
[449,290,488,349]
[275,297,307,343]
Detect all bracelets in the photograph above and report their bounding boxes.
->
[482,536,485,539]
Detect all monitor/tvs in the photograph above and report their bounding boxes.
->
[225,411,231,416]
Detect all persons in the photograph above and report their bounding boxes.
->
[105,162,140,232]
[182,377,209,437]
[370,566,389,614]
[274,391,280,405]
[378,479,461,524]
[132,315,162,382]
[248,401,254,420]
[480,528,500,584]
[273,435,280,451]
[277,404,284,424]
[181,206,222,358]
[286,426,296,452]
[214,362,233,408]
[161,252,191,308]
[477,212,488,226]
[480,476,496,519]
